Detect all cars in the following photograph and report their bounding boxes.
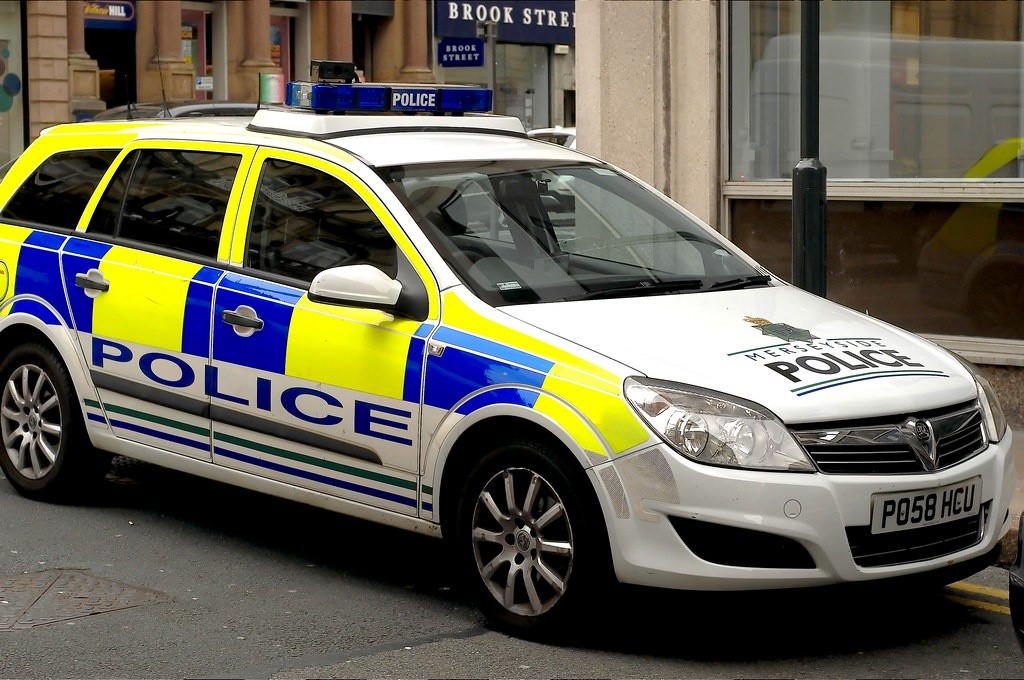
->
[498,126,576,207]
[0,61,1015,647]
[898,138,1024,327]
[0,99,286,186]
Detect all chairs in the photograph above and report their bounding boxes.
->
[272,186,373,280]
[411,185,513,306]
